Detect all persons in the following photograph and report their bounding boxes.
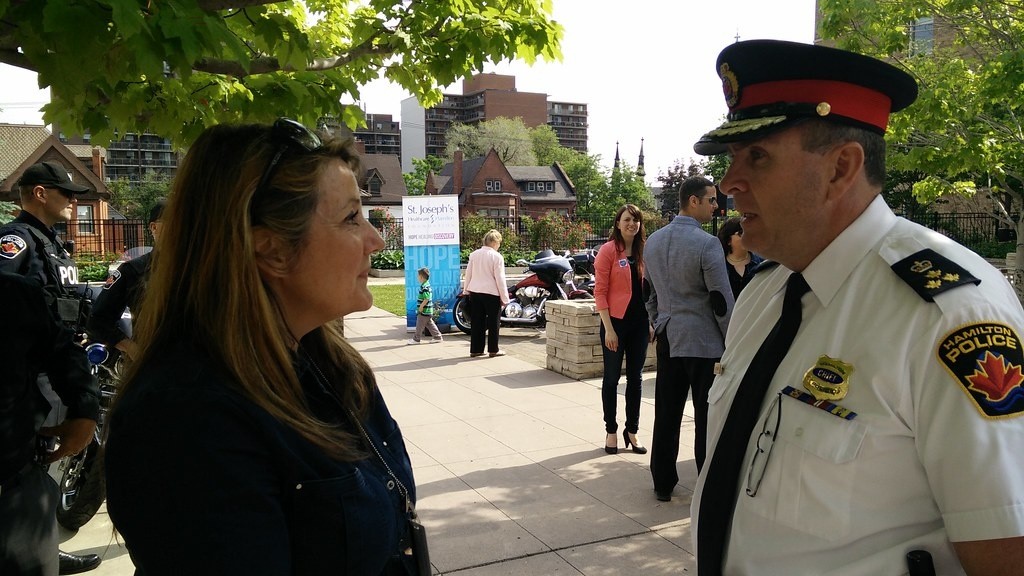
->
[718,218,764,301]
[0,162,165,576]
[102,122,432,576]
[463,230,509,357]
[595,204,654,455]
[642,176,735,501]
[407,268,443,344]
[690,39,1024,576]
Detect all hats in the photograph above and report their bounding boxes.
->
[693,39,919,156]
[17,161,89,195]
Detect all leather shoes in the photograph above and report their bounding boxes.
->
[59,549,102,575]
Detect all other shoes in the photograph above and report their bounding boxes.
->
[656,492,671,501]
[489,351,506,357]
[407,339,420,345]
[470,353,487,357]
[429,339,443,343]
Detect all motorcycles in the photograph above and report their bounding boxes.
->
[452,245,602,335]
[48,240,154,531]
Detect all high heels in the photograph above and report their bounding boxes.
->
[605,434,617,454]
[623,428,647,454]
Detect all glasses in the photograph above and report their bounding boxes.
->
[688,195,717,205]
[32,187,74,199]
[746,393,782,497]
[732,229,743,237]
[253,116,323,200]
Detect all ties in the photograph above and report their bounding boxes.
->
[697,271,813,576]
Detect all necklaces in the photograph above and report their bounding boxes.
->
[310,354,417,519]
